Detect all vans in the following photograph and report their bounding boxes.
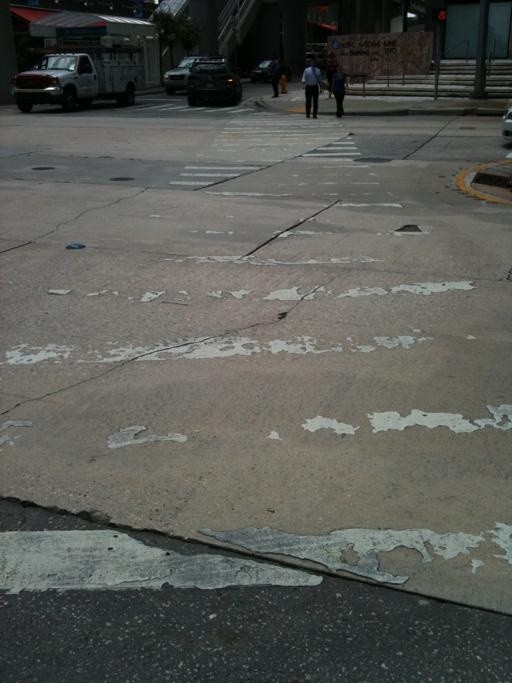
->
[187,57,243,107]
[162,55,212,95]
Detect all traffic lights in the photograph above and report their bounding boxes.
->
[432,4,447,22]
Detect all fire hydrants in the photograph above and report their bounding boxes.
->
[279,74,287,94]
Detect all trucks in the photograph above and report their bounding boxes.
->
[12,52,142,111]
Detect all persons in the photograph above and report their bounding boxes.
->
[301,56,324,119]
[266,52,280,97]
[323,50,339,97]
[329,62,346,118]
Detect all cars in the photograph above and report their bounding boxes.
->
[250,59,279,82]
